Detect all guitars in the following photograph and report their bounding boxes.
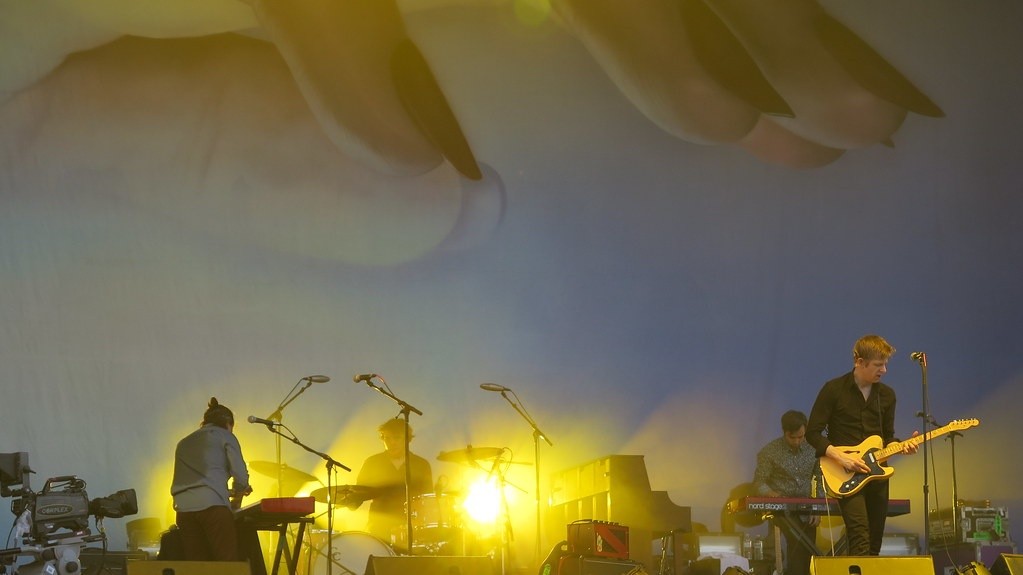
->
[820,416,981,498]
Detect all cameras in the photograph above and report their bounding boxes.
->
[12,472,138,545]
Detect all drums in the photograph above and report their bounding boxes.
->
[402,493,458,549]
[312,530,397,575]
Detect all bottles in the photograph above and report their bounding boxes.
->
[754,535,763,560]
[743,533,753,559]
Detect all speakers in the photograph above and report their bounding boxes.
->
[989,552,1023,575]
[809,554,934,575]
[363,554,495,575]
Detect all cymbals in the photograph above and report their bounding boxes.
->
[250,460,318,482]
[311,484,380,503]
[435,447,504,463]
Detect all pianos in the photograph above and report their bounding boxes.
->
[738,496,911,556]
[231,495,317,575]
[547,454,692,575]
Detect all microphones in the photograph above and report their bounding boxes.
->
[480,382,511,391]
[352,373,376,383]
[302,374,331,383]
[909,352,922,360]
[247,415,280,425]
[915,411,930,417]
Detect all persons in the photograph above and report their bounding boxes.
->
[0,0,947,267]
[356,418,433,556]
[804,334,920,575]
[753,409,828,575]
[171,397,250,562]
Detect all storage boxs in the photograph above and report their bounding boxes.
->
[933,540,1018,575]
